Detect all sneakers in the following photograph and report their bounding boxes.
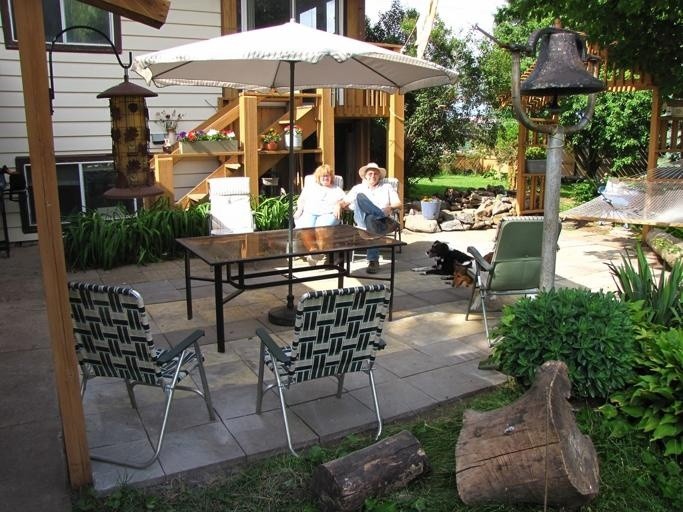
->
[386,220,399,233]
[368,261,379,273]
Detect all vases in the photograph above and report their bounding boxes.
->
[179,140,238,154]
[420,202,440,221]
[266,141,280,152]
[286,135,303,151]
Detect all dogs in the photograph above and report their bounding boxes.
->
[452,251,494,288]
[410,240,474,285]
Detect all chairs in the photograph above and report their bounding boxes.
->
[207,174,255,279]
[466,214,562,347]
[255,285,392,461]
[292,173,345,261]
[68,282,216,469]
[350,175,402,266]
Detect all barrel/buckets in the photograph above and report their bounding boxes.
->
[421,198,441,219]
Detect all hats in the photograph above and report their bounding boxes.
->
[359,162,386,180]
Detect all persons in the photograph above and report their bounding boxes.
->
[341,160,403,276]
[296,164,349,268]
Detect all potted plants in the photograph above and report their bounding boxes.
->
[156,108,186,148]
[522,144,549,175]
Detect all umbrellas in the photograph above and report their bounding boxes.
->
[129,20,457,328]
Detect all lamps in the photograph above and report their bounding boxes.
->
[49,24,167,202]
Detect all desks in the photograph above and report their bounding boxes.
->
[173,222,408,354]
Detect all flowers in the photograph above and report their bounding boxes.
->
[257,128,283,143]
[422,194,439,201]
[283,120,304,135]
[177,126,237,144]
